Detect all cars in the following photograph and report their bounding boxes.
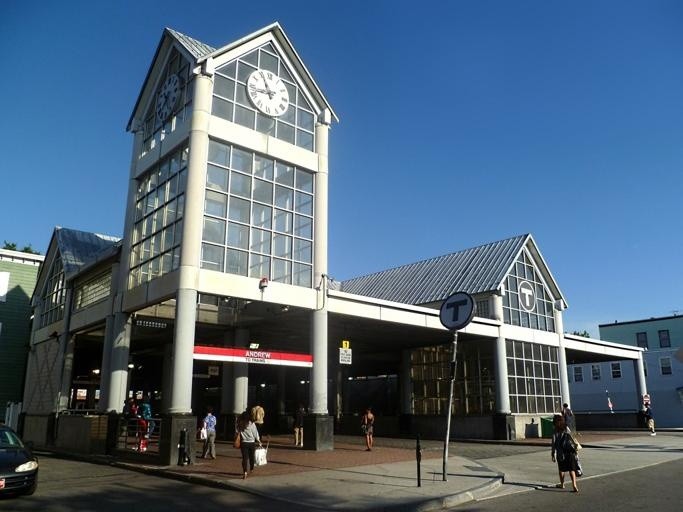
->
[0,422,38,495]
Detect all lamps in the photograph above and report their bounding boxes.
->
[47,331,62,344]
[259,278,268,290]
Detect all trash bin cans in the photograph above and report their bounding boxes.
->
[540,416,554,438]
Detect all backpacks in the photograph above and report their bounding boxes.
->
[253,407,261,421]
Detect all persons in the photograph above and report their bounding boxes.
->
[123,397,157,453]
[359,406,375,451]
[551,415,581,492]
[200,409,217,459]
[561,403,577,437]
[642,403,656,436]
[292,407,304,447]
[250,401,265,442]
[237,411,262,479]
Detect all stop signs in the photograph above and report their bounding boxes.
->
[643,396,651,405]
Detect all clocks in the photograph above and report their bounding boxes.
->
[246,70,289,118]
[156,73,182,122]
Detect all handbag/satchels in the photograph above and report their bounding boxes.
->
[196,422,207,440]
[359,424,368,436]
[253,446,268,467]
[233,431,241,449]
[554,432,578,452]
[574,455,583,477]
